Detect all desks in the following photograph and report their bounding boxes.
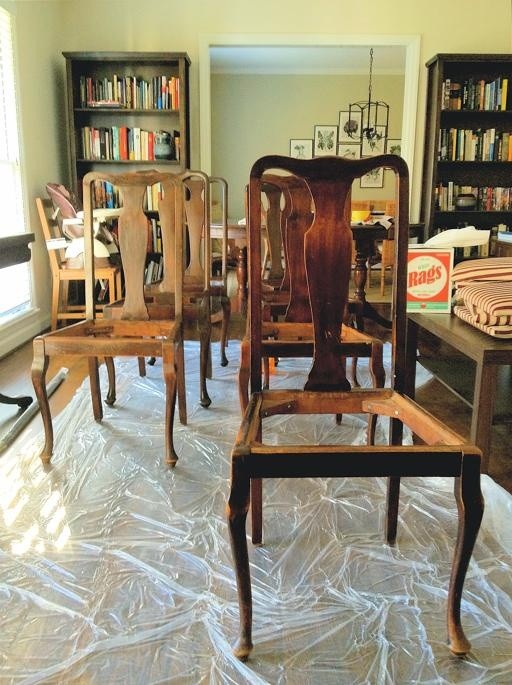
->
[411,311,510,480]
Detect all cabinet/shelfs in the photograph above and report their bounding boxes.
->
[421,55,512,257]
[61,52,191,305]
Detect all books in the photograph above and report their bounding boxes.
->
[431,66,511,269]
[71,70,186,305]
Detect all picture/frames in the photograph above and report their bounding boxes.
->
[290,112,401,188]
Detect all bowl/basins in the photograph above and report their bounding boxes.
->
[350,210,385,224]
